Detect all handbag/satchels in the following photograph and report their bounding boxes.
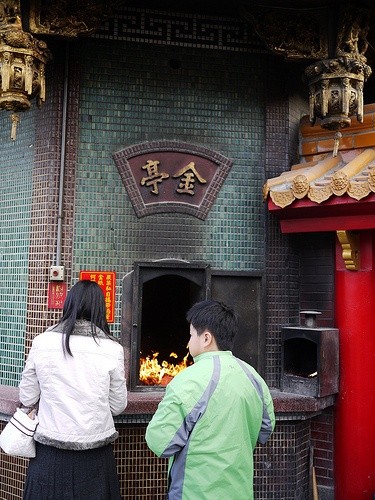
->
[0,397,39,457]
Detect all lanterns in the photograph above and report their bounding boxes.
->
[0,0,52,142]
[304,27,373,158]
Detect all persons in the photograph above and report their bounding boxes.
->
[145,299,275,500]
[19,280,127,500]
